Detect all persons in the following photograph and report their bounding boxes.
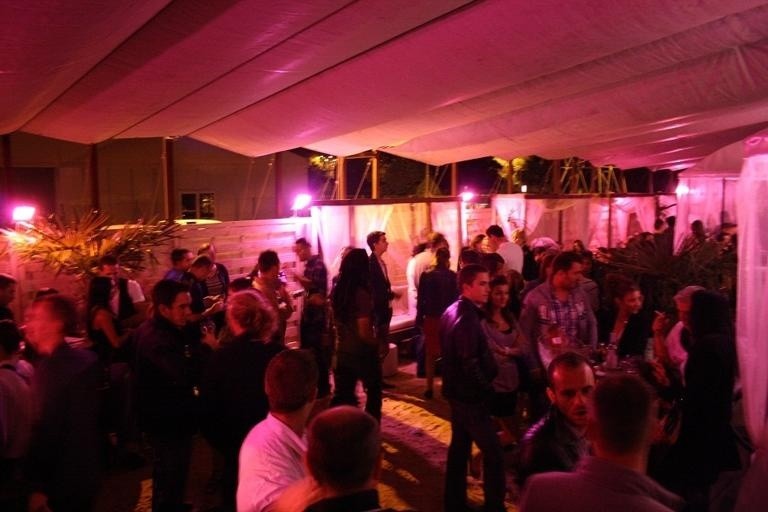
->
[3,230,403,511]
[404,213,767,511]
